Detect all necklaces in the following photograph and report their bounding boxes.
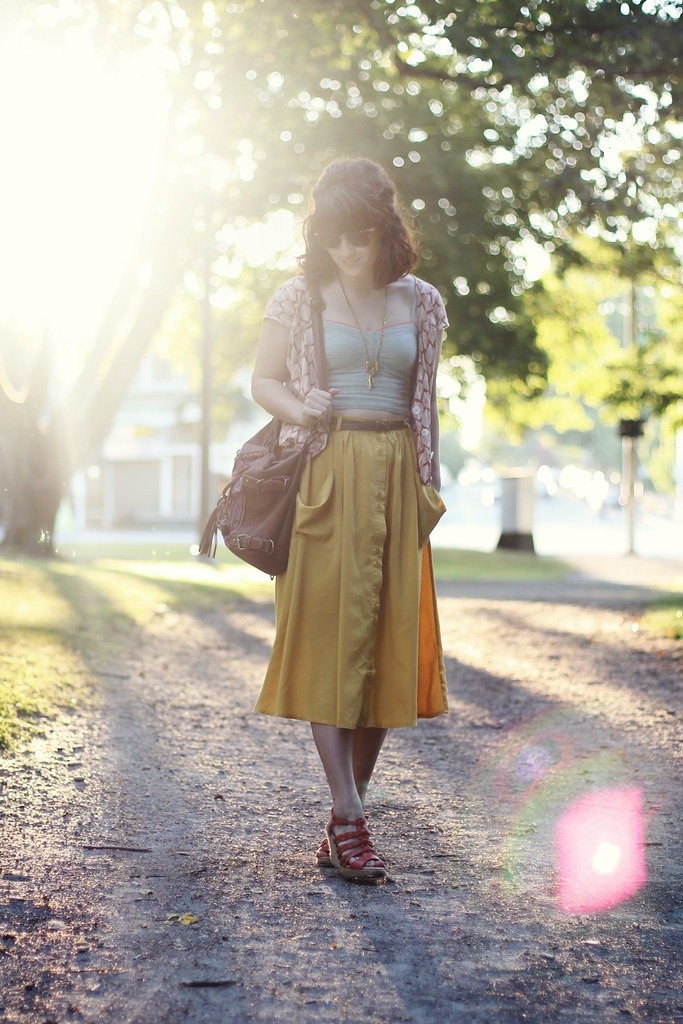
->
[337,272,388,390]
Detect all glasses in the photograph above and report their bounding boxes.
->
[313,224,378,249]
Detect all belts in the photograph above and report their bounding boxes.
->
[329,420,406,433]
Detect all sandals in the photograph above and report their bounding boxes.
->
[325,806,386,878]
[316,838,332,866]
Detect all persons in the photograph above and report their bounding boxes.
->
[251,158,448,879]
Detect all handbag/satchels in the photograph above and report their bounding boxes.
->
[197,419,308,575]
